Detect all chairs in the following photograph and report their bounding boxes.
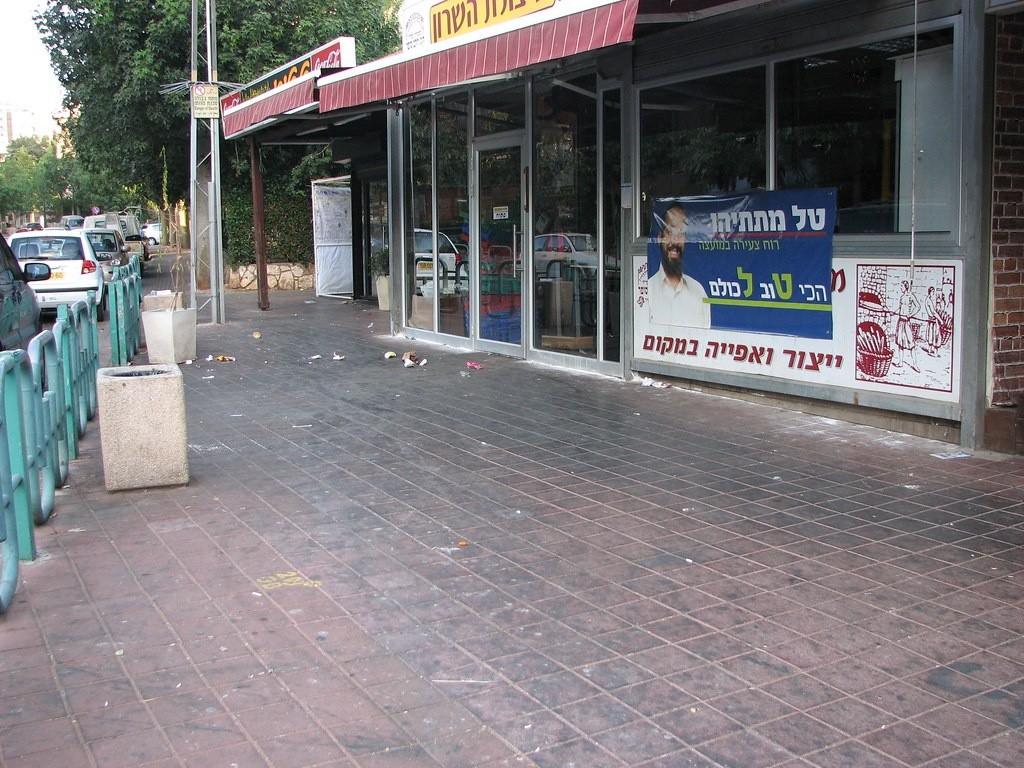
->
[61,242,81,258]
[101,238,113,250]
[19,244,38,257]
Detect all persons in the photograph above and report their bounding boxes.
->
[647,206,711,329]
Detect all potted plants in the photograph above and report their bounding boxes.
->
[126,147,197,365]
[367,246,390,311]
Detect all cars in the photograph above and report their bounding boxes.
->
[517,233,621,277]
[15,213,165,293]
[7,231,113,322]
[413,228,470,296]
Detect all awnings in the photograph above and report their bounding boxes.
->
[222,68,361,139]
[317,0,687,113]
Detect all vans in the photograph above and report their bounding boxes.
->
[0,231,53,391]
